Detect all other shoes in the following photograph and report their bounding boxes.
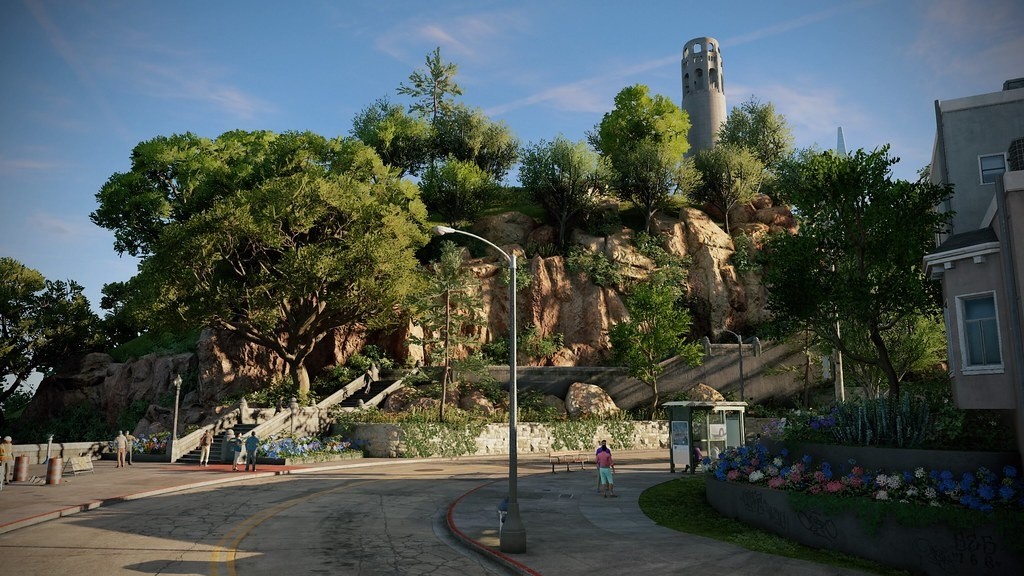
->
[604,495,607,497]
[610,495,618,498]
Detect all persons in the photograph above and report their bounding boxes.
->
[113,430,128,468]
[232,432,243,472]
[124,430,135,465]
[245,431,261,471]
[0,435,15,485]
[594,439,612,493]
[691,433,703,472]
[200,429,213,466]
[595,444,618,498]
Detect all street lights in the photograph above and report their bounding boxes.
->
[172,374,182,441]
[713,328,744,400]
[432,224,527,554]
